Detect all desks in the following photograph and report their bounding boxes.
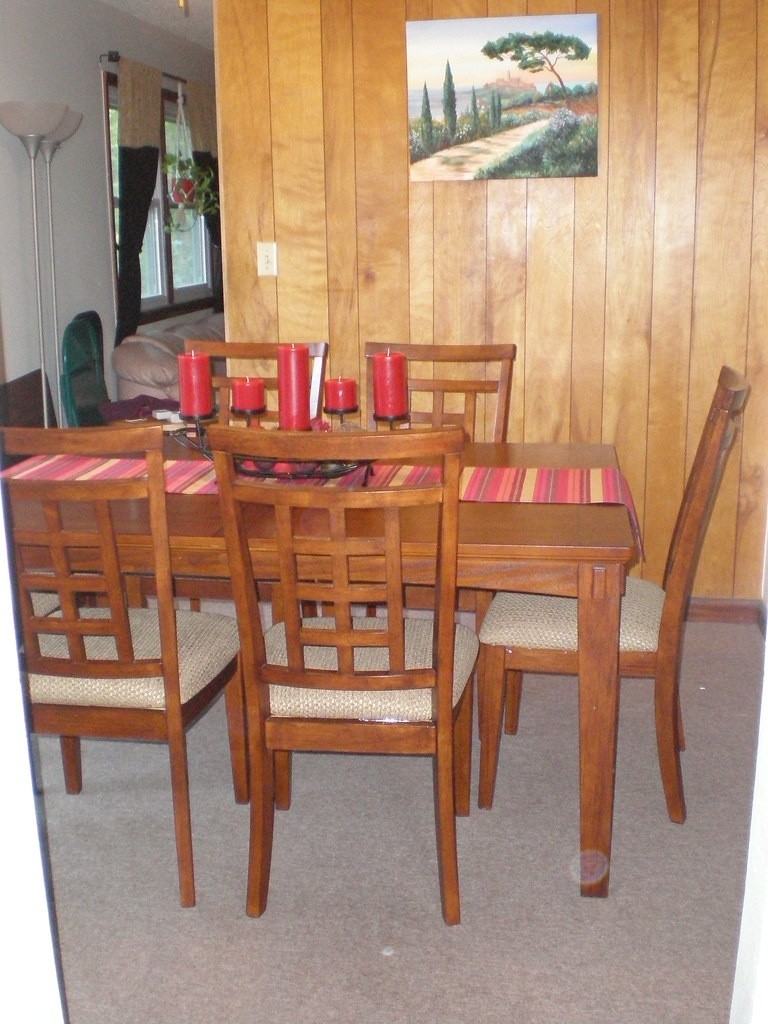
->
[0,442,645,899]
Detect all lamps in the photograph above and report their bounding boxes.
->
[0,102,84,429]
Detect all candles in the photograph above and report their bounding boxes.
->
[176,348,214,420]
[373,346,409,421]
[229,377,266,415]
[323,376,358,414]
[277,343,312,432]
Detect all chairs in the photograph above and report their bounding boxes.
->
[0,340,749,925]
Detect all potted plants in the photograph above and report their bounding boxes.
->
[160,151,218,232]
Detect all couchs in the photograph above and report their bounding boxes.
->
[112,311,227,403]
